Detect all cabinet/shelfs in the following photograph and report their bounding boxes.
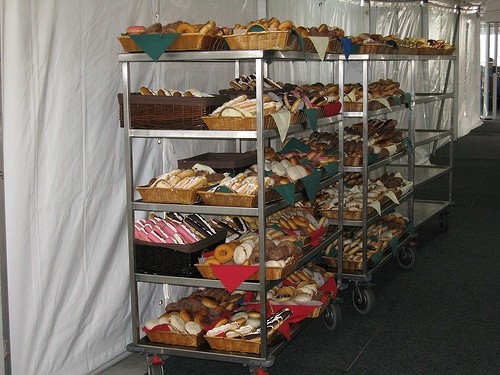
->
[118,50,457,375]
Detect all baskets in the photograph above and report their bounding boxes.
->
[209,25,225,50]
[244,272,337,318]
[118,93,230,130]
[318,237,382,274]
[203,303,294,354]
[266,207,328,258]
[315,181,402,221]
[290,36,456,54]
[396,178,413,195]
[177,151,257,178]
[352,119,398,134]
[310,139,405,168]
[136,178,208,205]
[197,180,299,208]
[222,23,289,51]
[290,93,325,124]
[354,226,408,261]
[248,161,339,192]
[194,249,303,280]
[201,107,295,130]
[337,93,405,112]
[117,33,210,52]
[142,302,209,347]
[134,227,227,277]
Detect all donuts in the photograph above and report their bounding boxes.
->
[203,204,327,267]
[124,20,231,35]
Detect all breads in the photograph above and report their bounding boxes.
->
[264,131,340,189]
[343,78,403,101]
[145,287,261,338]
[266,263,335,302]
[323,212,407,262]
[344,32,454,51]
[368,117,404,153]
[209,164,259,195]
[135,85,194,98]
[225,16,344,38]
[212,72,339,117]
[133,213,216,245]
[294,172,403,212]
[343,125,363,159]
[147,167,225,191]
